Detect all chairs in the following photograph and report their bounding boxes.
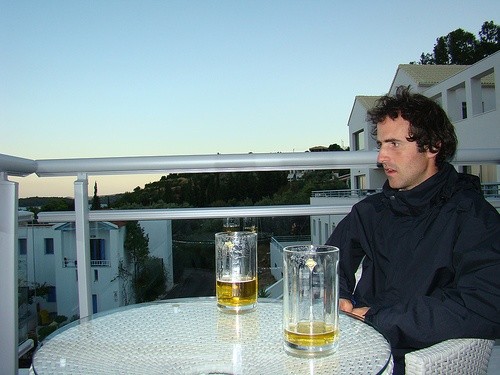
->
[352,264,495,375]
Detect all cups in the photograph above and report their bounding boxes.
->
[282,245,340,357]
[215,232,258,314]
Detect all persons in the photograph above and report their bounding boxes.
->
[325,84,500,375]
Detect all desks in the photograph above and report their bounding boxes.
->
[28,296,394,375]
